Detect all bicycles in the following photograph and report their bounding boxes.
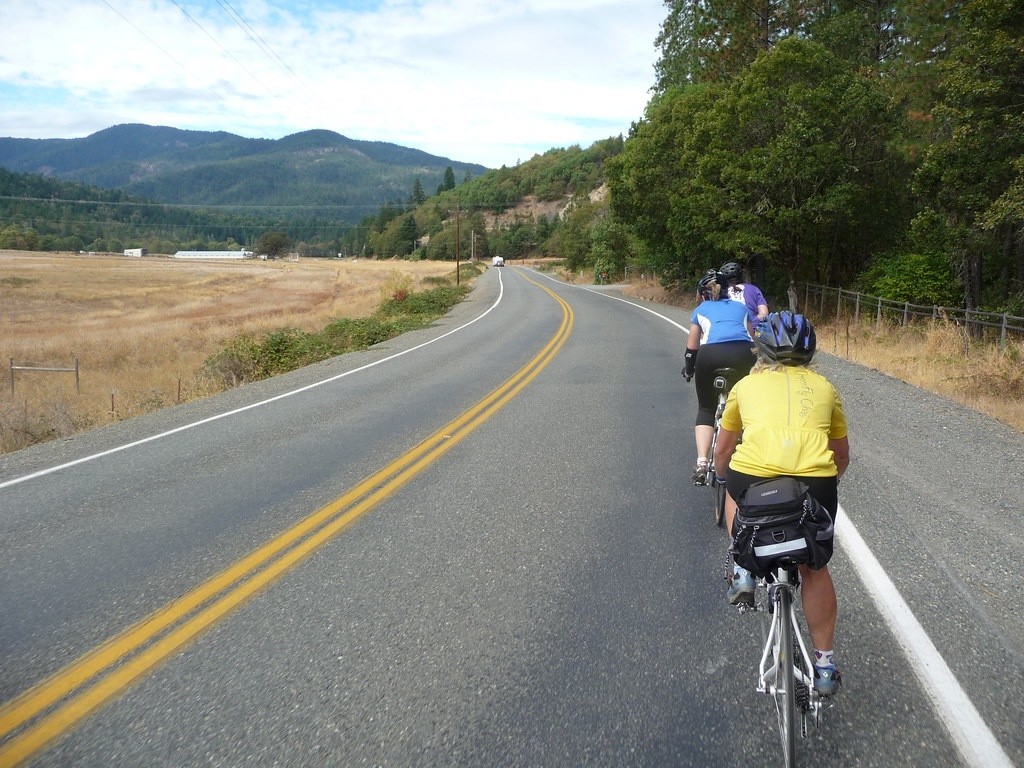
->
[735,553,830,768]
[680,362,745,527]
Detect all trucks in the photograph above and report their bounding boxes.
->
[492,256,504,267]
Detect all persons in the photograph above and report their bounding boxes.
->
[681,263,768,483]
[713,310,851,696]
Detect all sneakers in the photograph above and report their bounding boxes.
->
[814,665,841,695]
[726,567,755,606]
[691,464,707,483]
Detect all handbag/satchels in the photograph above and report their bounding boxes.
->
[731,478,834,579]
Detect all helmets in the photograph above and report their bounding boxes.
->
[697,271,728,297]
[755,311,816,360]
[719,262,743,284]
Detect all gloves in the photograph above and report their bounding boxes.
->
[681,368,695,381]
